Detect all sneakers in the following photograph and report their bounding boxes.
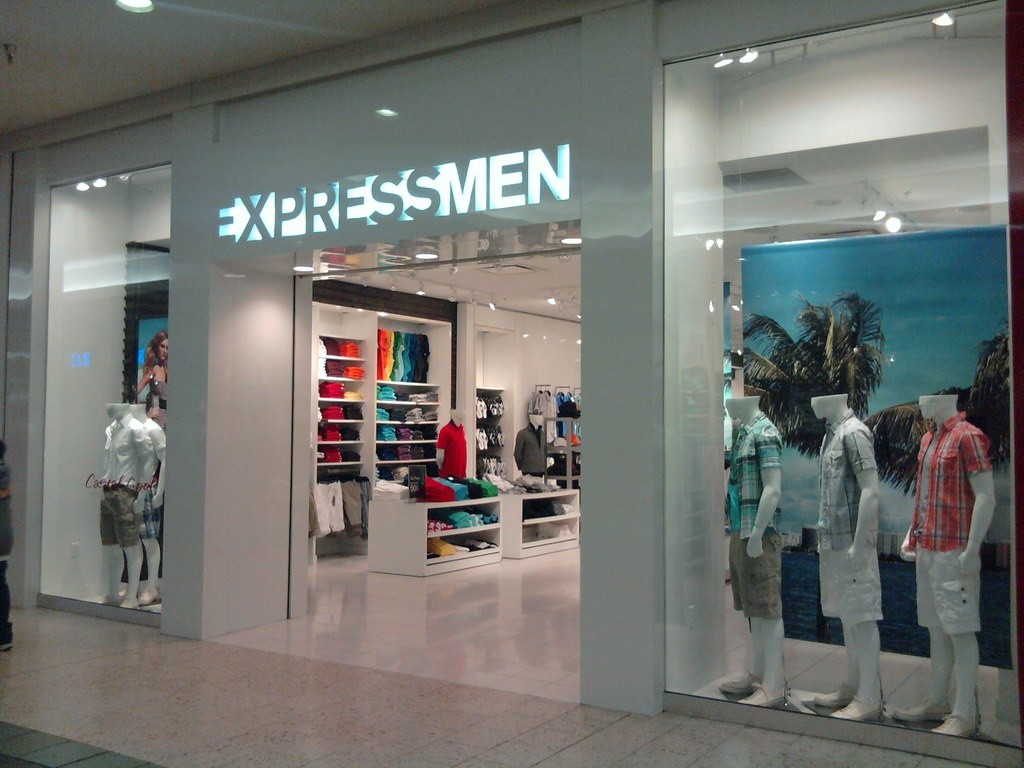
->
[738,683,784,708]
[930,713,974,738]
[814,685,856,707]
[719,669,761,694]
[893,702,951,722]
[830,694,880,721]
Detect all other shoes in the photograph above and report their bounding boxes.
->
[0,623,13,651]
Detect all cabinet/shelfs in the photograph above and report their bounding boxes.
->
[310,300,452,484]
[494,488,581,561]
[540,418,581,492]
[367,494,503,578]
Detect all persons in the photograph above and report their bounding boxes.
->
[100,403,168,610]
[436,409,468,478]
[720,396,786,710]
[810,393,885,722]
[515,415,546,478]
[138,330,169,409]
[893,394,995,737]
[0,441,14,651]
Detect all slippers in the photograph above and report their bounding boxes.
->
[478,453,507,479]
[476,423,504,450]
[477,396,503,420]
